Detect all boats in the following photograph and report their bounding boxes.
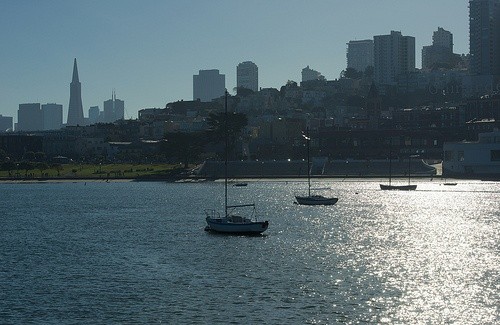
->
[231,183,248,186]
[443,183,458,185]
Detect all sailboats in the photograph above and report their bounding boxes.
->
[204,89,269,235]
[294,125,339,206]
[379,136,419,190]
